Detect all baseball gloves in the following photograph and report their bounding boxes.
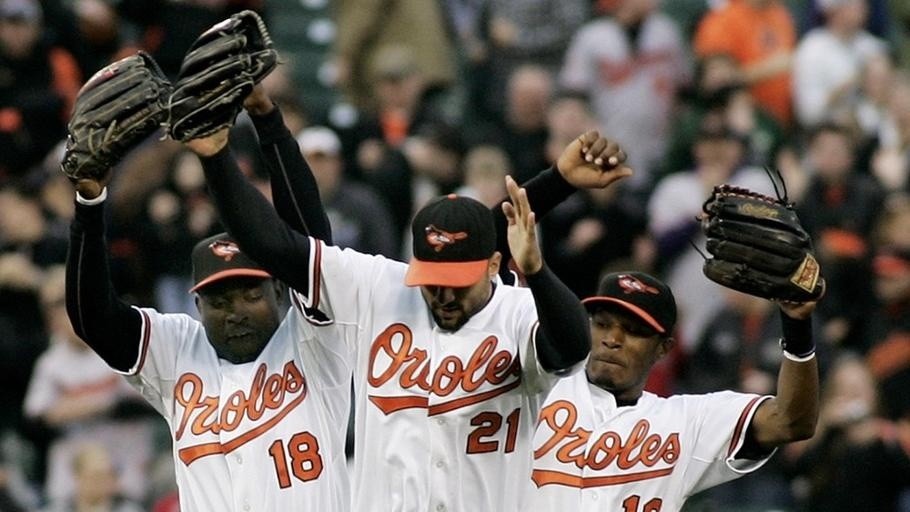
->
[155,10,284,144]
[683,163,828,306]
[59,49,174,183]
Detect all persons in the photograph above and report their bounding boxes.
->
[0,0,910,512]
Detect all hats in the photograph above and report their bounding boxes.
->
[405,192,497,287]
[189,231,270,294]
[581,270,676,332]
